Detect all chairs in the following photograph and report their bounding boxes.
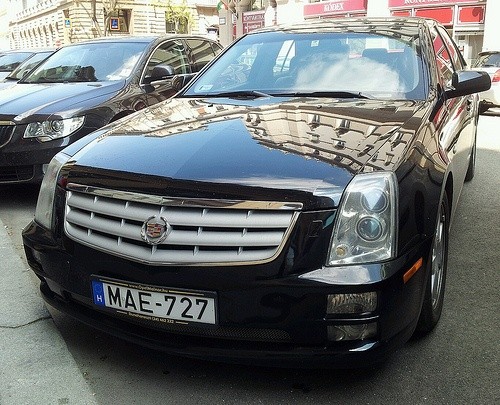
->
[273,55,311,89]
[359,48,409,81]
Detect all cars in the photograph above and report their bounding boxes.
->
[0,35,223,190]
[20,16,491,371]
[472,50,499,114]
